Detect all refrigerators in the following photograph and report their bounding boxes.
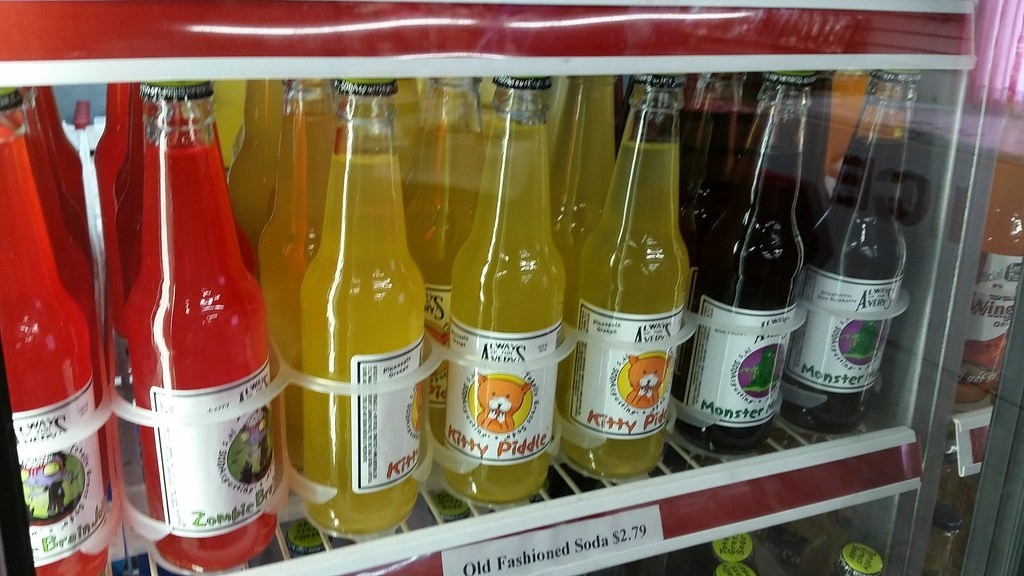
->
[0,0,1024,576]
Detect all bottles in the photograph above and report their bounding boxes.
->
[0,69,1024,576]
